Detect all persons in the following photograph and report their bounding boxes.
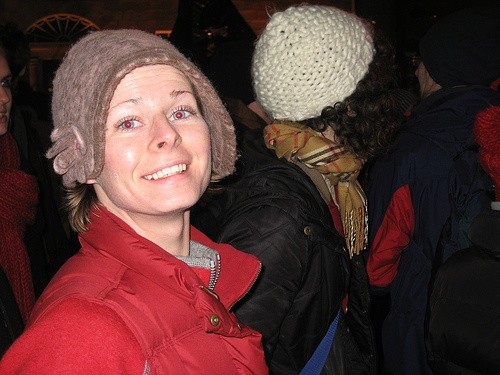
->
[204,2,403,375]
[366,6,500,375]
[0,24,72,356]
[0,30,269,375]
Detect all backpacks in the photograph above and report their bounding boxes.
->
[402,104,499,375]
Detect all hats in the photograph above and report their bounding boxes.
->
[251,4,375,118]
[419,4,500,85]
[45,28,235,182]
